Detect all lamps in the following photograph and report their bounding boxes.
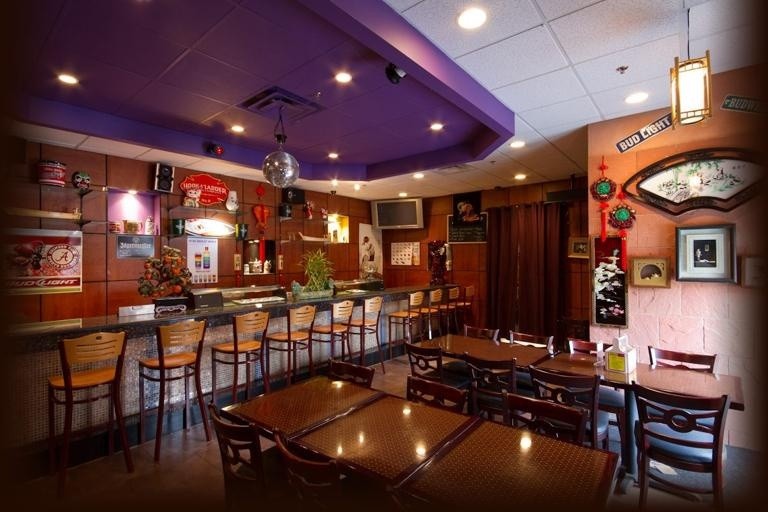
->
[385,65,407,85]
[243,86,324,189]
[203,140,225,157]
[669,9,714,128]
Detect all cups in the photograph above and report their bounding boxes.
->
[194,254,202,268]
[107,222,121,233]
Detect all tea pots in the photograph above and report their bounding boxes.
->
[123,220,143,232]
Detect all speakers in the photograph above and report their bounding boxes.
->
[151,163,175,193]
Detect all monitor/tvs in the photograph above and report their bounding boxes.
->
[370,197,425,232]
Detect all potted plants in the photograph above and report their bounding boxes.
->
[291,248,336,301]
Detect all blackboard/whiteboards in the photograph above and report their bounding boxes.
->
[446,212,489,244]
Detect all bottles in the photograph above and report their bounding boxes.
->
[145,216,154,235]
[244,264,249,274]
[203,246,211,270]
[226,191,240,211]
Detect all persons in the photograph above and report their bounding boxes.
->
[455,200,480,226]
[362,236,376,261]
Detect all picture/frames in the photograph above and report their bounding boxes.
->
[622,146,765,289]
[591,233,628,329]
[566,236,591,259]
